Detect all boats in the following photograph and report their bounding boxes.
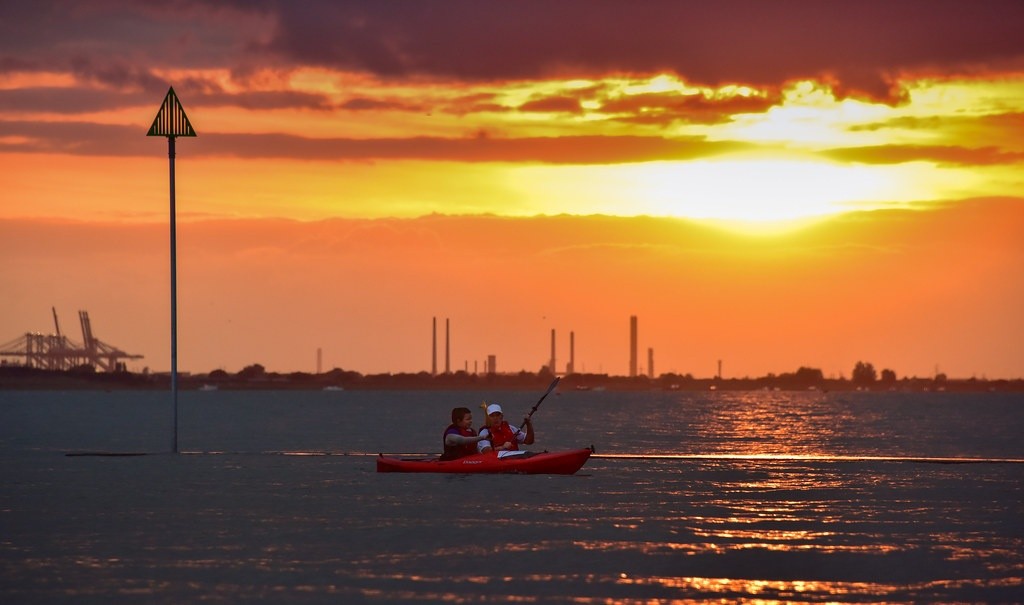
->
[374,446,593,474]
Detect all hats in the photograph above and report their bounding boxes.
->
[486,404,503,416]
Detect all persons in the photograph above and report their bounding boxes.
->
[478,403,535,454]
[443,408,494,459]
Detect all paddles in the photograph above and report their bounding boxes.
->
[502,376,561,450]
[478,400,494,450]
